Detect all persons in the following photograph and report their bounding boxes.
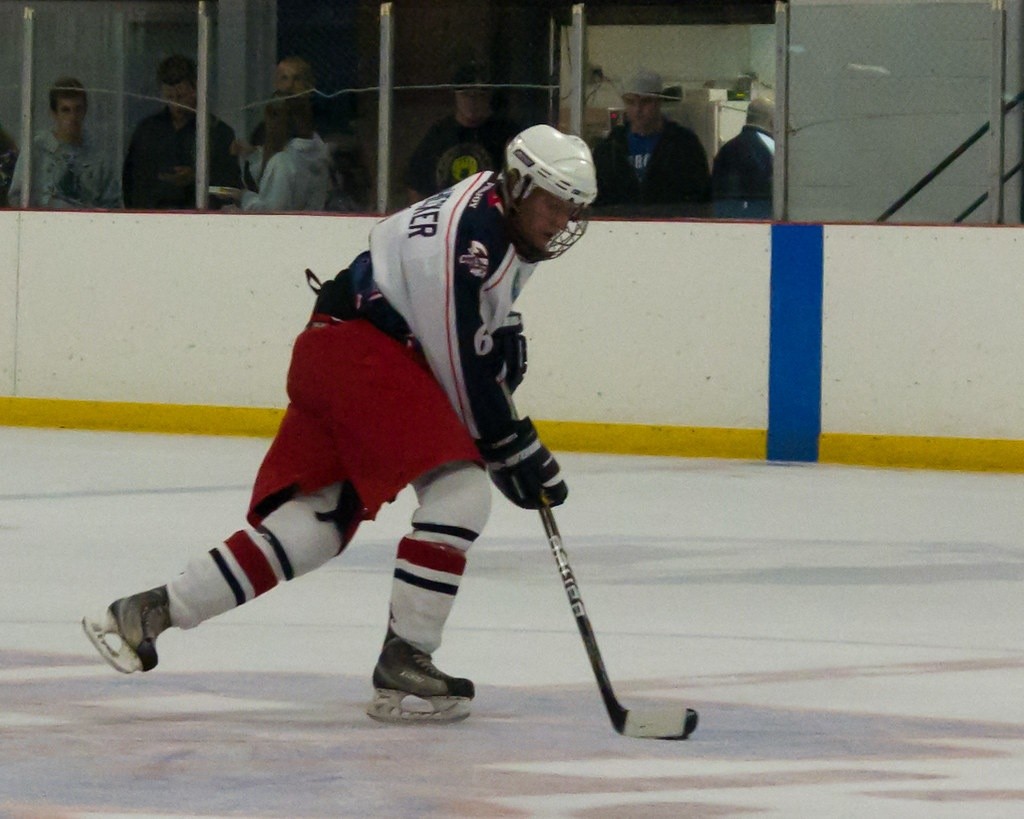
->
[235,97,338,217]
[119,59,241,212]
[248,59,369,212]
[709,94,776,219]
[404,72,515,215]
[80,114,602,726]
[596,72,711,219]
[3,79,122,214]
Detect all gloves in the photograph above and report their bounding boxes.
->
[476,414,568,509]
[489,309,529,397]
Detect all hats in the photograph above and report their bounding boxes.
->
[619,65,663,97]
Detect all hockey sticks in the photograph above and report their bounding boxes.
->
[501,379,699,741]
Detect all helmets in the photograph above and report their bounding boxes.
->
[504,123,598,262]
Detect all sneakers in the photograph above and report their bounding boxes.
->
[109,583,171,672]
[373,627,476,701]
